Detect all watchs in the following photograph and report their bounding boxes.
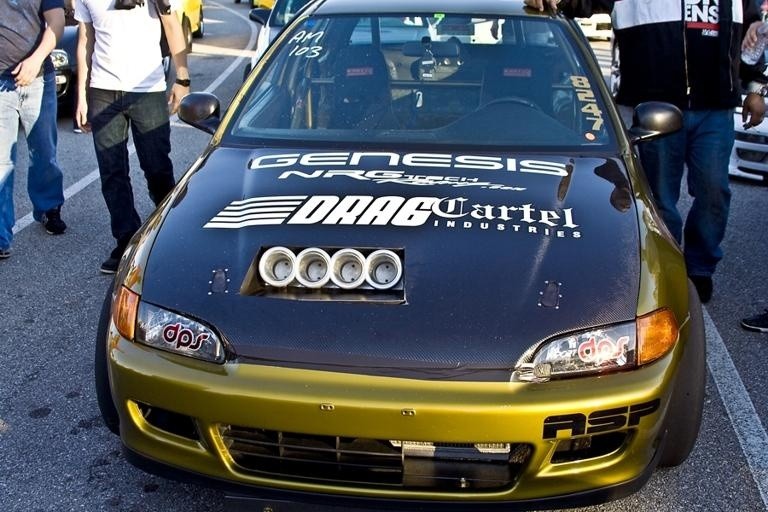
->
[748,85,768,97]
[176,78,191,87]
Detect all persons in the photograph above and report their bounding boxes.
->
[594,159,633,214]
[71,1,192,275]
[739,20,768,332]
[0,1,67,256]
[523,1,768,303]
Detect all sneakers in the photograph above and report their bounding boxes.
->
[690,275,712,302]
[99,258,119,274]
[739,313,767,333]
[40,208,66,235]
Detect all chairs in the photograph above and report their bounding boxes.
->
[480,47,553,115]
[326,43,400,130]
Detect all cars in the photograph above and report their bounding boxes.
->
[44,8,83,108]
[94,1,708,511]
[574,12,767,184]
[244,0,443,83]
[174,0,205,52]
